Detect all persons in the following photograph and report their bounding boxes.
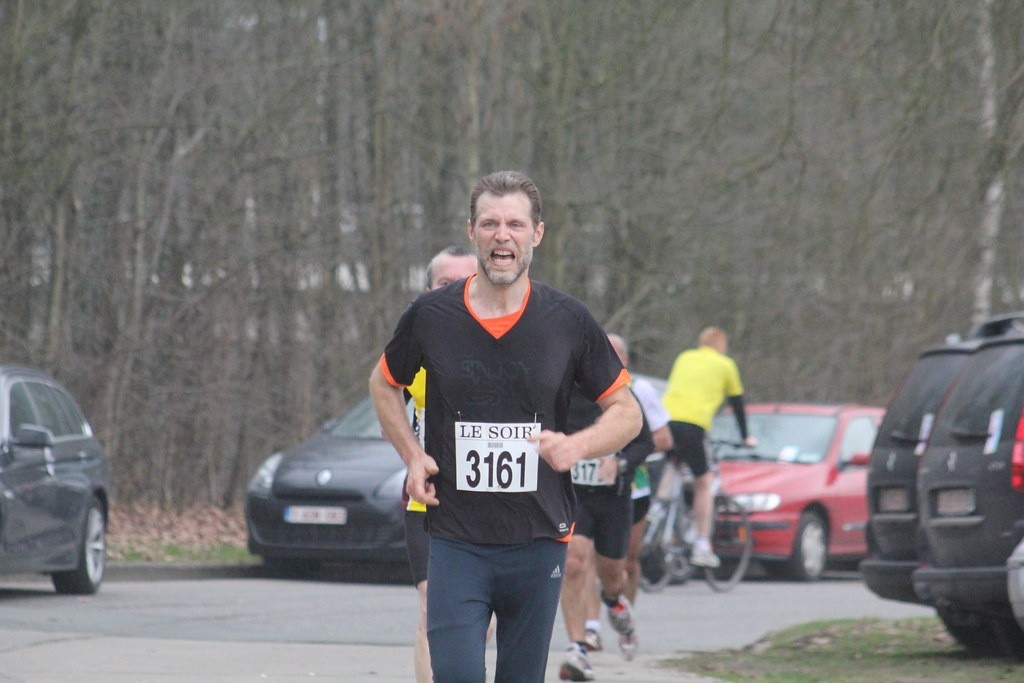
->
[382,246,478,683]
[648,325,757,568]
[585,331,672,651]
[367,170,644,683]
[559,389,656,681]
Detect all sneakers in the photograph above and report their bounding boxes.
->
[558,643,594,681]
[618,630,639,661]
[582,628,601,651]
[596,578,635,635]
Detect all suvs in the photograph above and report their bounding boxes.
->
[860,311,1024,664]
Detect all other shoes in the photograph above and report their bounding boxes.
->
[645,501,667,522]
[689,545,721,569]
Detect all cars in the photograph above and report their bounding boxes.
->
[243,391,424,578]
[671,402,886,584]
[0,365,109,595]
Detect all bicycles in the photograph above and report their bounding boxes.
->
[630,438,756,593]
